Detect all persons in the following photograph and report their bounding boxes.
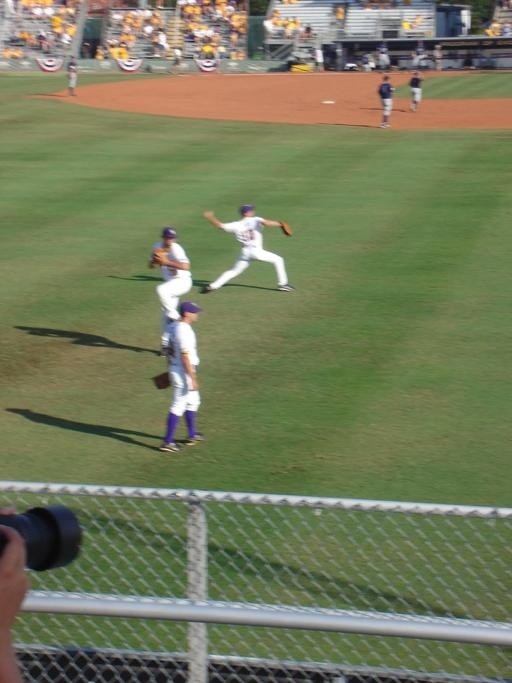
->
[66,56,78,97]
[335,6,344,20]
[311,48,324,66]
[147,226,193,357]
[486,1,511,36]
[264,11,314,37]
[363,0,409,9]
[158,301,207,453]
[409,71,425,112]
[0,506,29,683]
[96,0,248,58]
[403,14,422,29]
[377,75,398,129]
[346,45,474,71]
[201,203,296,293]
[1,0,82,56]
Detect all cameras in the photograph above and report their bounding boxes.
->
[0,501,85,572]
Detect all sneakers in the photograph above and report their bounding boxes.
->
[279,285,295,291]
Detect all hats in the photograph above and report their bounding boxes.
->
[165,227,176,238]
[242,205,253,212]
[181,303,202,312]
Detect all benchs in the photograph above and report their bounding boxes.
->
[0,0,512,62]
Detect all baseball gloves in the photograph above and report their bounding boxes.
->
[152,248,167,263]
[153,372,170,389]
[280,221,290,236]
